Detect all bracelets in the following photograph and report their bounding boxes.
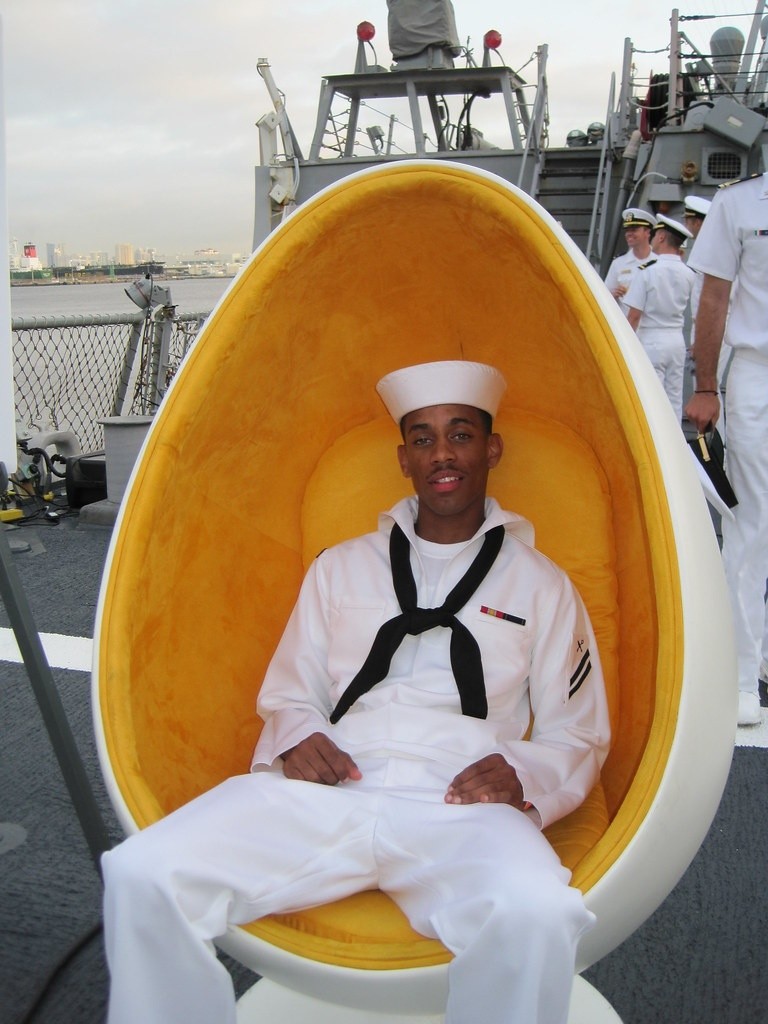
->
[694,390,719,396]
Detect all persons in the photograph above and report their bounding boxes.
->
[603,170,768,726]
[100,361,611,1024]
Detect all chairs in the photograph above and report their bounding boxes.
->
[91,158,740,1024]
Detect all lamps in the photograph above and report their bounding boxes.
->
[124,277,154,309]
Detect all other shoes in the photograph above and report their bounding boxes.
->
[737,690,761,726]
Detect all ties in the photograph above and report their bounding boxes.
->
[330,521,507,728]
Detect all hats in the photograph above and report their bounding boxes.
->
[687,421,738,524]
[682,196,713,221]
[622,208,657,230]
[376,360,507,423]
[654,213,695,239]
